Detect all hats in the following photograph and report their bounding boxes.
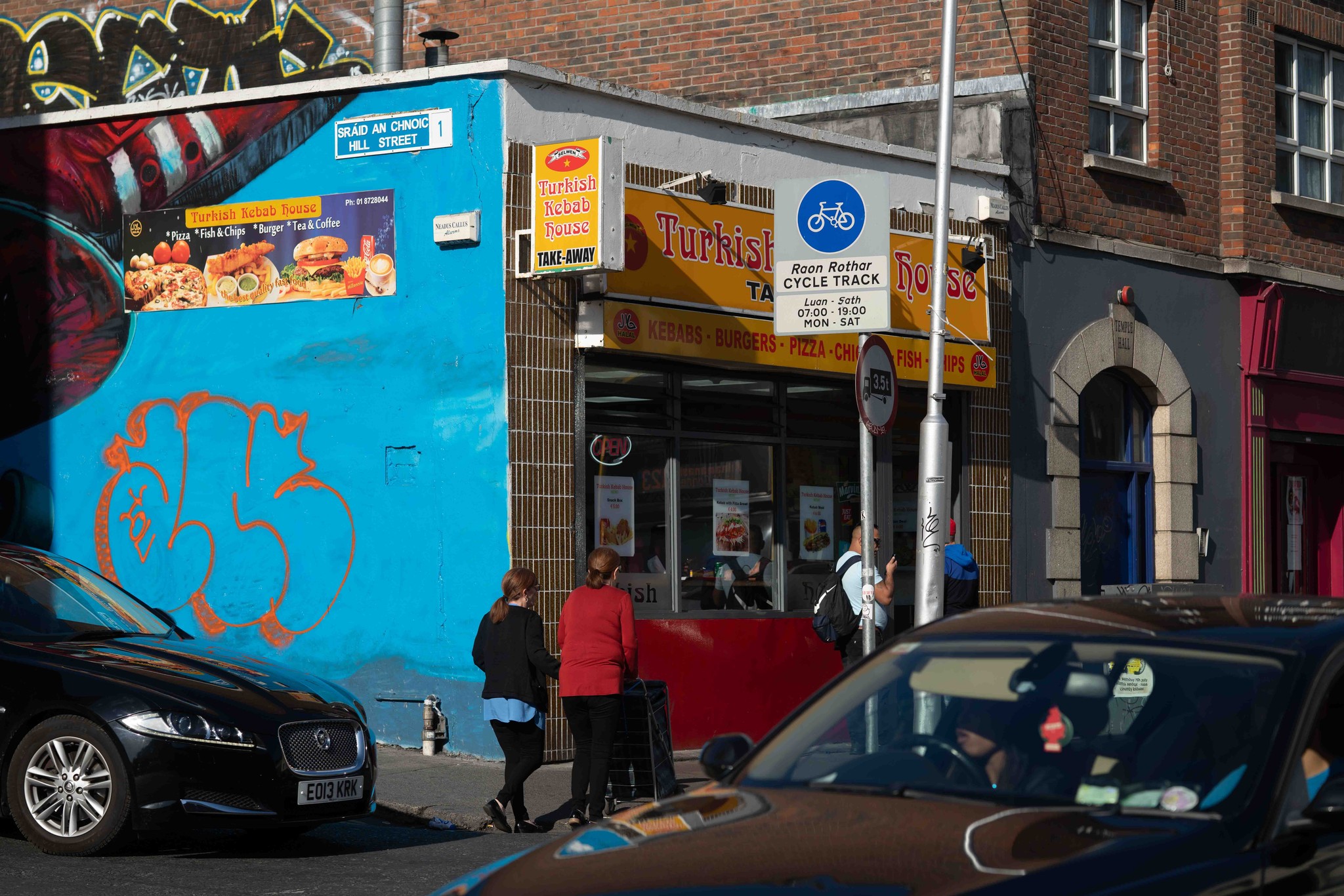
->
[950,518,956,535]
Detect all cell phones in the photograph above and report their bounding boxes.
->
[884,554,896,576]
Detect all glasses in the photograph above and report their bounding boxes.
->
[529,584,541,591]
[615,565,621,572]
[874,538,881,545]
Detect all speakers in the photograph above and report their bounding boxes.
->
[611,679,678,800]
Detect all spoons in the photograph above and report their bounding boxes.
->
[365,278,384,294]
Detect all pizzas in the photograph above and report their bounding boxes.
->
[125,263,208,311]
[718,516,747,545]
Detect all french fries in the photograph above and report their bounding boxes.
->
[310,282,347,297]
[206,259,271,303]
[341,255,366,278]
[605,524,633,545]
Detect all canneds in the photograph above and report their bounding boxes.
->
[360,235,375,266]
[818,519,826,533]
[600,518,610,545]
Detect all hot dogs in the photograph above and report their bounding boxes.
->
[803,532,830,552]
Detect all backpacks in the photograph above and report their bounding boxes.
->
[727,556,773,610]
[811,555,862,643]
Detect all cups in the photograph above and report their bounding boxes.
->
[365,253,393,285]
[216,275,238,303]
[237,273,259,299]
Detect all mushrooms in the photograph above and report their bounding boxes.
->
[130,253,155,271]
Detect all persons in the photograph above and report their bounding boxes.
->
[556,547,641,826]
[942,689,1071,806]
[827,523,898,673]
[943,517,981,620]
[713,524,776,611]
[471,567,562,833]
[1196,684,1344,823]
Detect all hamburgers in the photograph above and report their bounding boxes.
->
[280,236,348,292]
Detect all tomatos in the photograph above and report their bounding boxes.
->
[171,240,190,264]
[153,241,172,264]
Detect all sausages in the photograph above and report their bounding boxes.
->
[804,519,817,533]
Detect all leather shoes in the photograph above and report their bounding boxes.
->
[514,820,544,834]
[569,810,588,826]
[589,821,595,825]
[483,798,513,833]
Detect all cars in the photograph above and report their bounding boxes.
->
[428,593,1344,896]
[628,493,773,579]
[0,540,378,856]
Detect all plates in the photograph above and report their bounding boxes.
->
[365,267,396,296]
[203,253,286,308]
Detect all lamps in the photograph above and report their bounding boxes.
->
[948,234,986,273]
[1117,286,1134,305]
[656,169,727,205]
